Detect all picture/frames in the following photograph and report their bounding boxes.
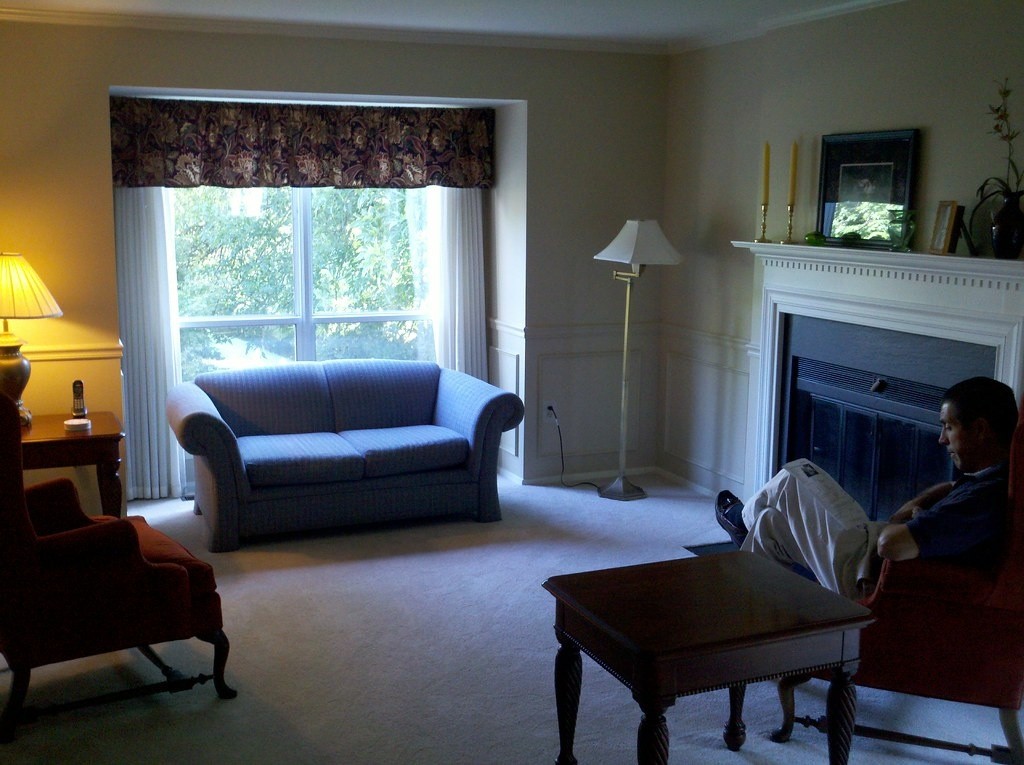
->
[815,128,925,251]
[928,198,962,256]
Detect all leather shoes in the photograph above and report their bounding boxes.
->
[715,490,748,548]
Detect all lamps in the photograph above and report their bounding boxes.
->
[0,247,66,429]
[591,213,690,502]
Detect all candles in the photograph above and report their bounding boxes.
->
[787,136,799,207]
[760,139,772,205]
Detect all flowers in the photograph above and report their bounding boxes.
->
[969,74,1024,239]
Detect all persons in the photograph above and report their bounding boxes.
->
[716,376,1024,597]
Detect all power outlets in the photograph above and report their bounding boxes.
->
[543,398,559,425]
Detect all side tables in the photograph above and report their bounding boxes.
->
[538,548,881,765]
[22,405,128,520]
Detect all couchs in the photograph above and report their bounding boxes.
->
[763,393,1024,765]
[0,392,238,746]
[159,358,530,553]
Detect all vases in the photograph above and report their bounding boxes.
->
[991,188,1024,259]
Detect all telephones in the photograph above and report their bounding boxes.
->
[71,379,87,419]
